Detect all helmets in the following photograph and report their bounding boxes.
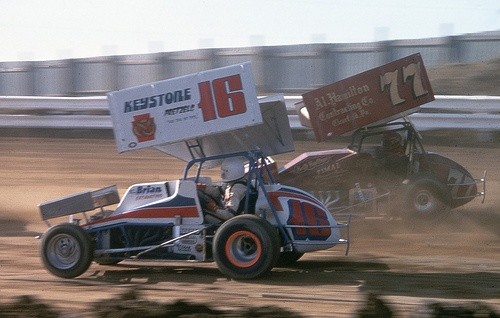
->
[381,133,401,154]
[220,158,245,183]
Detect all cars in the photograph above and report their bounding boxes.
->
[39,149,351,283]
[244,121,486,224]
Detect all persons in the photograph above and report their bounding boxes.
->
[195,157,250,224]
[375,132,414,185]
